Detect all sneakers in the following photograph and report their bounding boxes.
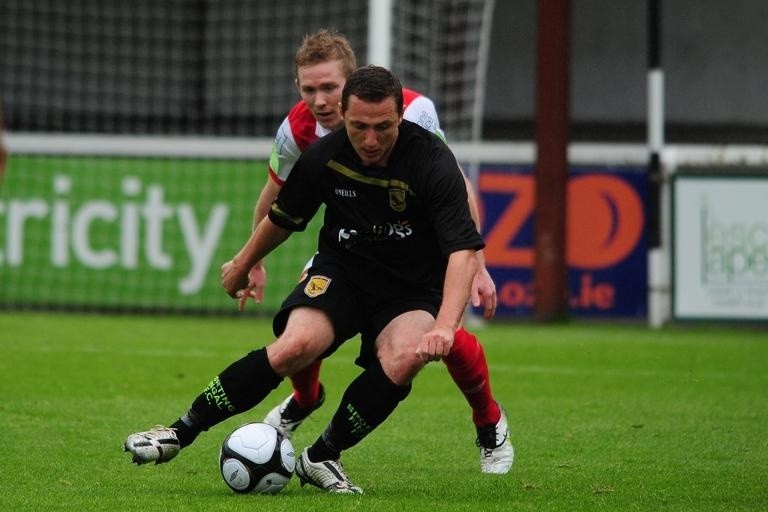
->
[475,405,514,474]
[264,383,326,438]
[124,424,181,466]
[295,446,365,494]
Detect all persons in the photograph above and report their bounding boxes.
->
[122,63,486,495]
[237,26,515,475]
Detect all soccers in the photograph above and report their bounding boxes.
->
[219,422,297,495]
